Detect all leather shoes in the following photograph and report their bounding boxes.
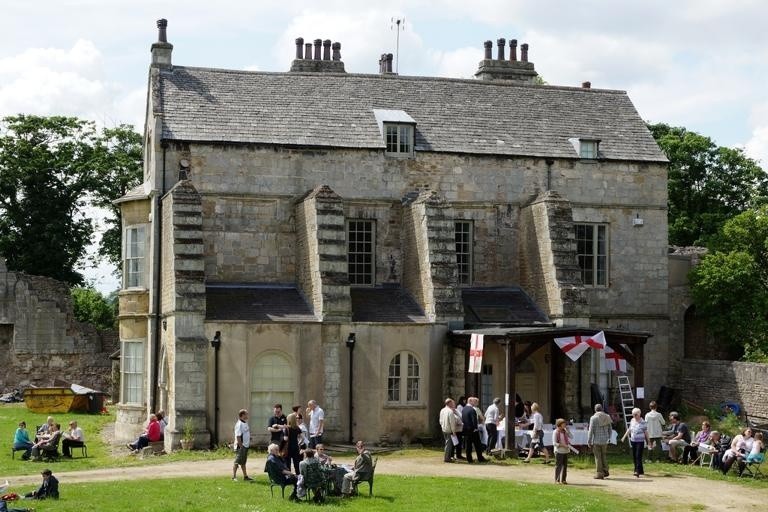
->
[543,461,552,465]
[522,460,530,464]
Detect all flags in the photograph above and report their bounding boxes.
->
[468,333,484,373]
[553,330,607,362]
[600,344,627,373]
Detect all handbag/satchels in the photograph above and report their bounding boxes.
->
[531,432,541,443]
[277,424,290,458]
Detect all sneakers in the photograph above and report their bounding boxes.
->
[244,477,252,481]
[232,478,238,481]
[127,444,134,451]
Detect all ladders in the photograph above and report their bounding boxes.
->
[619,375,637,448]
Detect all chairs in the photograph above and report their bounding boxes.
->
[303,449,322,469]
[41,435,61,462]
[699,431,718,468]
[353,457,379,496]
[681,431,695,463]
[304,465,327,505]
[266,463,284,500]
[740,448,766,480]
[38,425,60,431]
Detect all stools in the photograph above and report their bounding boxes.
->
[69,445,88,458]
[13,448,17,460]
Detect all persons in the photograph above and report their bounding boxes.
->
[341,441,373,494]
[264,400,333,503]
[439,393,551,463]
[125,410,167,452]
[13,417,85,462]
[588,403,612,479]
[17,469,60,500]
[644,400,765,477]
[553,418,574,485]
[620,407,653,478]
[231,408,252,481]
